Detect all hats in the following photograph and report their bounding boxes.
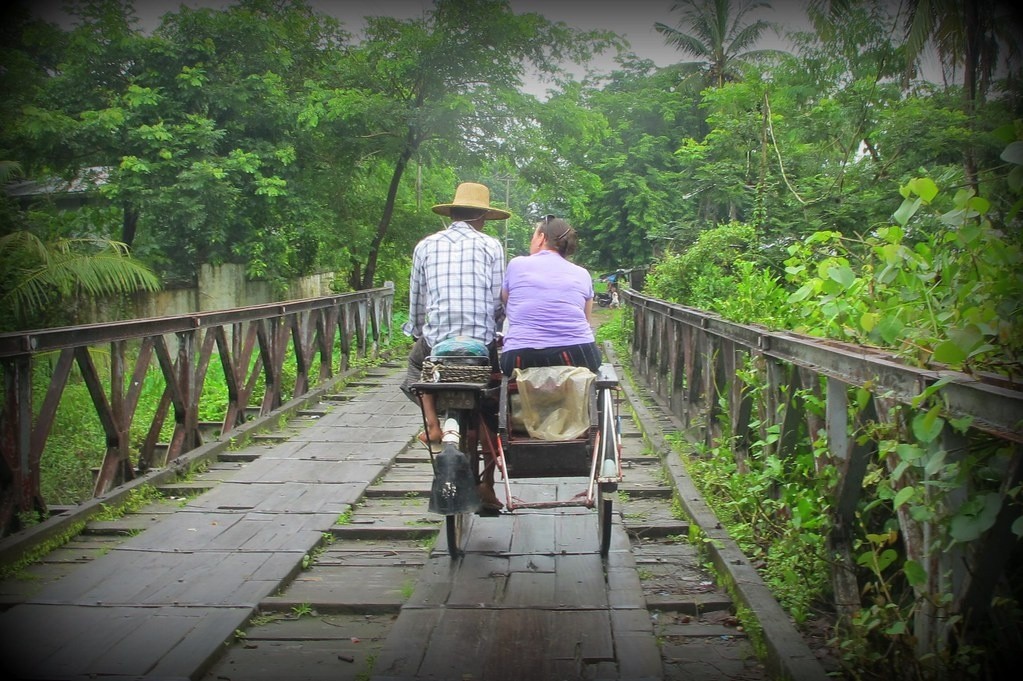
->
[432,182,511,221]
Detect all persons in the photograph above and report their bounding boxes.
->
[497,215,602,377]
[399,181,503,512]
[605,277,621,309]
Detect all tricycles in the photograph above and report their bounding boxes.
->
[413,352,622,557]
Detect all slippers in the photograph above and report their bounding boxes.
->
[476,500,503,516]
[418,431,442,453]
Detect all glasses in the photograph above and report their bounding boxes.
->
[540,213,554,242]
